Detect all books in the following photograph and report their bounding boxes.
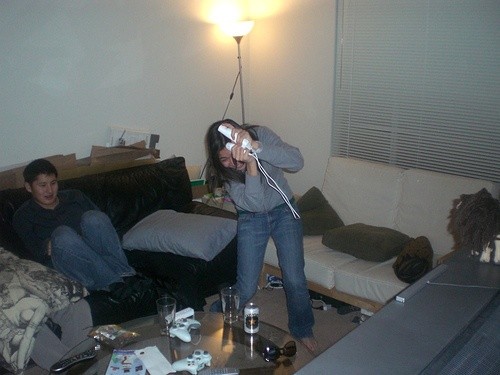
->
[106,350,146,375]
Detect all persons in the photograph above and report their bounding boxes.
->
[205,119,321,354]
[11,160,156,315]
[29,298,94,372]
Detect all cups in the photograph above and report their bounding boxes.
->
[155,295,179,338]
[219,285,240,326]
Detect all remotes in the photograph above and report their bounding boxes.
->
[49,346,98,373]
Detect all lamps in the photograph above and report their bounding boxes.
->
[227,21,254,125]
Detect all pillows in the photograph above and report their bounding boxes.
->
[123,209,237,262]
[322,223,410,262]
[296,186,345,235]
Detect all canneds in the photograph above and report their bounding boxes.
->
[244,303,260,335]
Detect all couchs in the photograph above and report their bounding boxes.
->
[1,157,238,324]
[251,156,496,313]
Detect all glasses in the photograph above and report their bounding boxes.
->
[263,341,296,362]
